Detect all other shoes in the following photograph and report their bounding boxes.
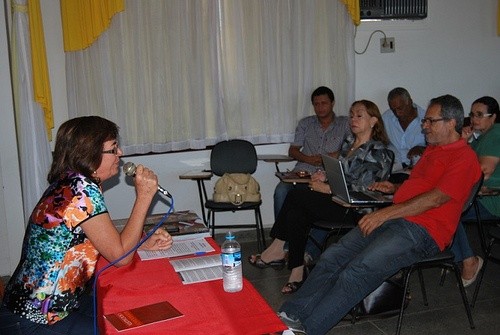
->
[280,330,307,335]
[462,256,483,287]
[275,312,304,332]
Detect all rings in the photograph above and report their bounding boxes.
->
[311,188,313,190]
[159,230,163,234]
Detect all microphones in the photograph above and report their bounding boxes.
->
[123,162,173,198]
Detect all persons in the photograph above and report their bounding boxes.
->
[382,87,427,169]
[450,95,500,287]
[0,116,173,335]
[248,99,395,294]
[276,95,481,335]
[273,86,357,267]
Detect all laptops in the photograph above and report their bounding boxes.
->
[322,157,394,206]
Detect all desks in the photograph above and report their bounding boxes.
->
[179,155,296,228]
[92,210,296,335]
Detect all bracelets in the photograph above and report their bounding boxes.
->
[329,189,333,194]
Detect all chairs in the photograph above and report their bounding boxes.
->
[205,138,265,254]
[300,166,500,335]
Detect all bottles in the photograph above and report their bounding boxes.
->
[221,230,243,293]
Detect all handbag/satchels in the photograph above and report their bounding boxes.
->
[213,173,261,205]
[339,270,410,321]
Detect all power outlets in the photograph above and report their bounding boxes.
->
[380,37,396,54]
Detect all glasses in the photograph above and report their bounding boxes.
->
[102,145,118,155]
[468,113,492,118]
[422,118,449,125]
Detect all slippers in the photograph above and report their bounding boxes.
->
[282,268,307,295]
[249,254,284,271]
